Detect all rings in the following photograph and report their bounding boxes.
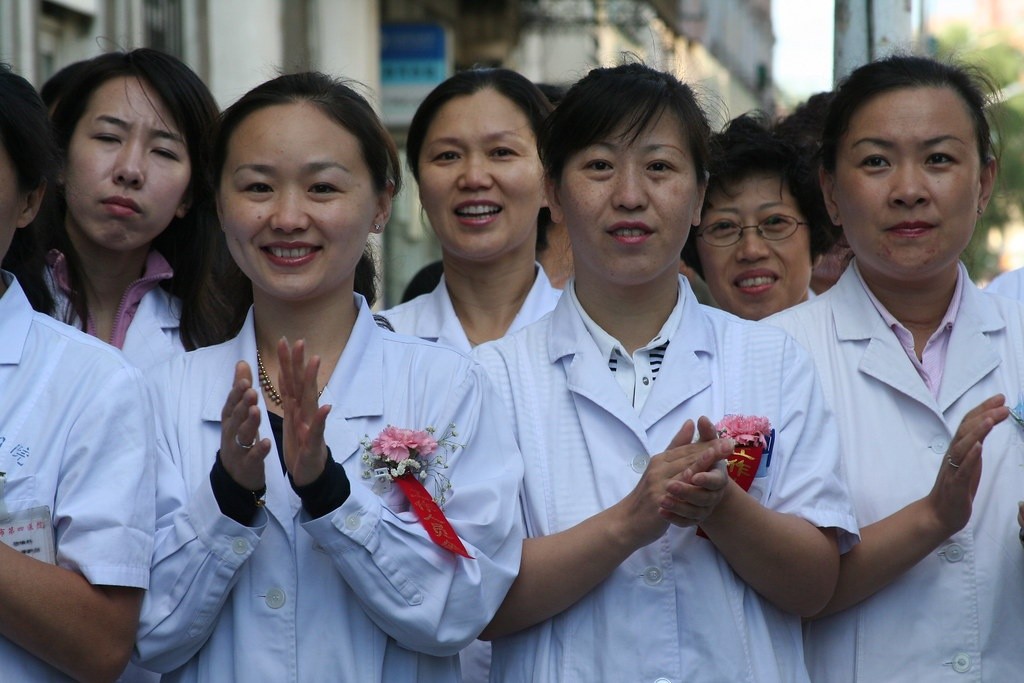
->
[236,435,257,450]
[947,455,959,468]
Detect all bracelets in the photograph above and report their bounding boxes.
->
[255,498,265,506]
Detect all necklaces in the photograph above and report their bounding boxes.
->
[256,361,280,405]
[255,349,323,409]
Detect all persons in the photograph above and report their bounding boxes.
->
[0,45,1024,683]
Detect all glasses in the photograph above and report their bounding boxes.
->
[696,213,812,247]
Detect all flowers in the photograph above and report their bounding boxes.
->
[713,410,772,449]
[359,421,467,513]
